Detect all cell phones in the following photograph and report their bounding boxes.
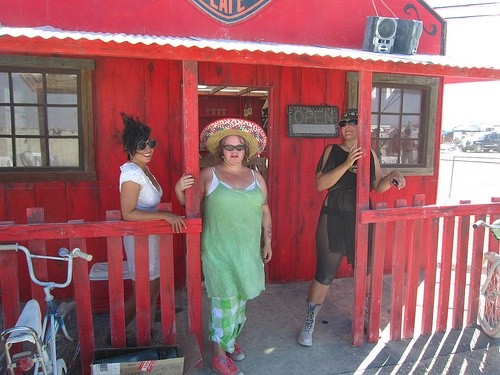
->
[391,178,398,186]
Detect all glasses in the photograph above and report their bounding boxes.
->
[338,119,357,127]
[224,145,245,151]
[137,139,156,151]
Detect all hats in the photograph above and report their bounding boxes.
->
[200,118,267,159]
[343,109,358,118]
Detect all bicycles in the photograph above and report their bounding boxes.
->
[472,219,500,339]
[0,243,93,375]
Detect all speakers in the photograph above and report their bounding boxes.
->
[394,19,423,55]
[360,15,399,53]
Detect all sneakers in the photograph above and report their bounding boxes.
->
[228,341,244,361]
[210,355,244,375]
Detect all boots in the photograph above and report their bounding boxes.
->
[365,294,381,338]
[298,304,323,347]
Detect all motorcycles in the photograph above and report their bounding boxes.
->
[447,137,462,151]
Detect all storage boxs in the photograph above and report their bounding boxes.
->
[89,344,185,375]
[89,260,161,315]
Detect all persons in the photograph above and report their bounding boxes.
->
[400,129,415,164]
[103,115,187,344]
[297,109,406,348]
[370,128,383,166]
[175,117,273,375]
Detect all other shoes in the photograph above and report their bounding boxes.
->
[104,331,159,345]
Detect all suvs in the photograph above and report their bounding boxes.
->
[461,131,500,153]
